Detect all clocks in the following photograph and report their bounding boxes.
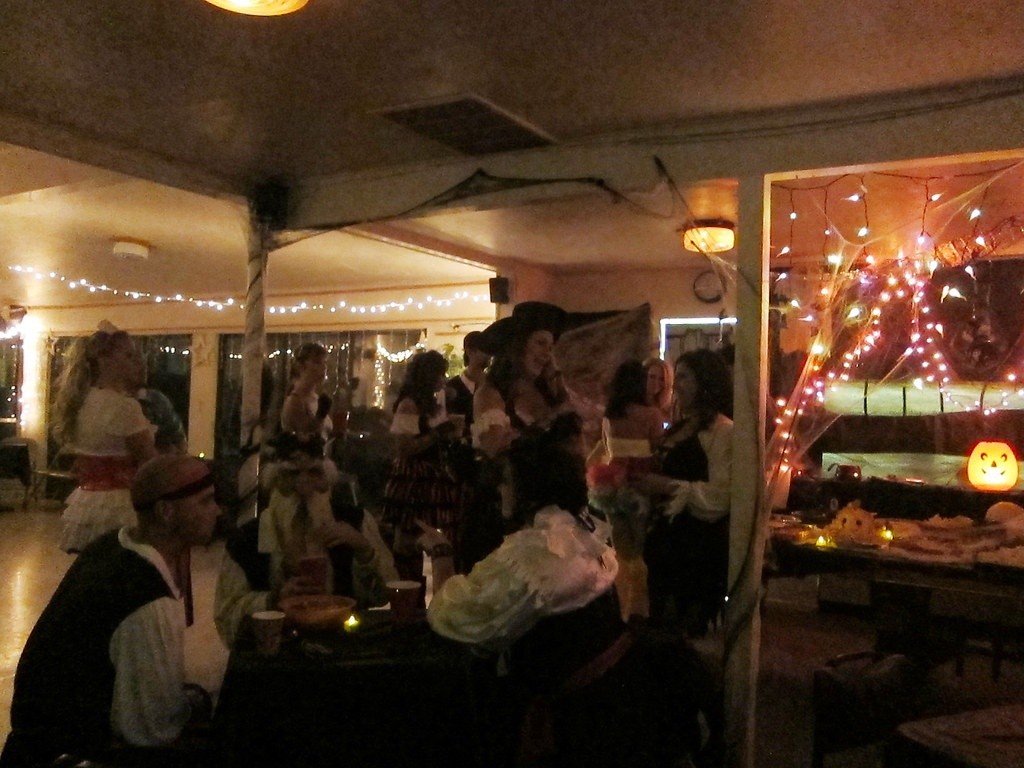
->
[693,270,727,303]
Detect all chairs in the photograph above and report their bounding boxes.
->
[811,650,924,768]
[21,445,79,512]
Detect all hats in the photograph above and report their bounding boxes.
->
[474,300,571,355]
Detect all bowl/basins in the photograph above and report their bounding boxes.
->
[277,593,356,631]
[833,465,862,481]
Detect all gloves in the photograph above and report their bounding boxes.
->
[317,393,332,419]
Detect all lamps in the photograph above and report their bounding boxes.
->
[966,434,1020,493]
[684,218,735,253]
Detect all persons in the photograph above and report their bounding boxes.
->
[214,432,400,652]
[390,351,465,582]
[416,432,622,768]
[133,343,188,456]
[474,301,600,466]
[644,360,668,408]
[588,359,662,625]
[444,331,491,456]
[281,343,349,455]
[629,349,733,768]
[55,320,155,551]
[334,445,403,546]
[0,457,221,768]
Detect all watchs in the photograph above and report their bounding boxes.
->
[427,543,453,559]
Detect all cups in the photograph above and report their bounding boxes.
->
[296,556,330,589]
[385,580,422,623]
[251,610,286,657]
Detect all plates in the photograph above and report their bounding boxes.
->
[766,512,816,540]
[917,518,974,531]
[915,536,1002,552]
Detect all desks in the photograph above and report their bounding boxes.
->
[894,700,1024,768]
[0,440,33,513]
[766,538,1023,683]
[204,610,510,768]
